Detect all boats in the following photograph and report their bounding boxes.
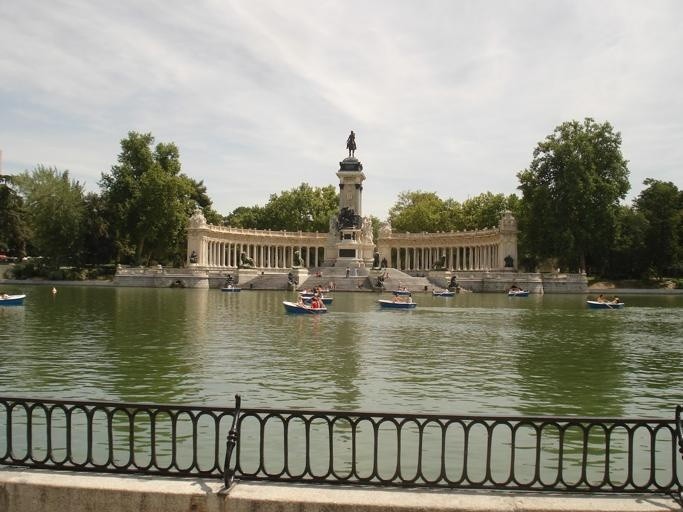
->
[220,281,242,293]
[433,290,456,297]
[376,299,417,310]
[280,286,335,314]
[1,293,25,304]
[585,299,628,310]
[506,288,529,297]
[391,286,412,296]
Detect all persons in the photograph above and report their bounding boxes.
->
[346,130,356,149]
[381,257,387,267]
[504,255,513,267]
[443,289,448,293]
[391,294,412,303]
[228,284,231,288]
[432,289,435,293]
[316,270,323,277]
[190,251,197,263]
[296,281,336,308]
[398,286,407,290]
[597,295,620,303]
[346,267,350,278]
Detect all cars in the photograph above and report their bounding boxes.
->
[0,253,46,267]
